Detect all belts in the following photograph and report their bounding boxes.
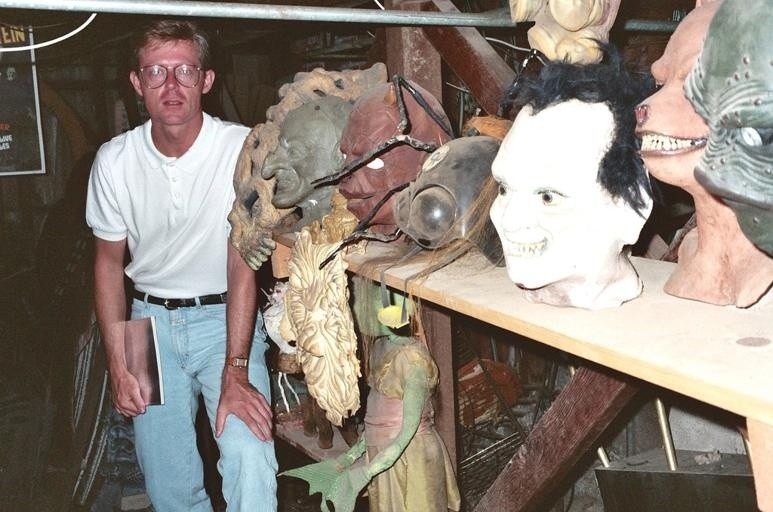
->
[131,289,227,311]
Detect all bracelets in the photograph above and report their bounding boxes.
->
[224,356,249,368]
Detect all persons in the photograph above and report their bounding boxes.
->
[489,33,665,311]
[81,18,281,512]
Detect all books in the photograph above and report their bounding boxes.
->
[122,317,166,405]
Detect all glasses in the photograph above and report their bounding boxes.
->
[137,62,205,90]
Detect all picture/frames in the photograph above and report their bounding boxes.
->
[0,25,48,178]
